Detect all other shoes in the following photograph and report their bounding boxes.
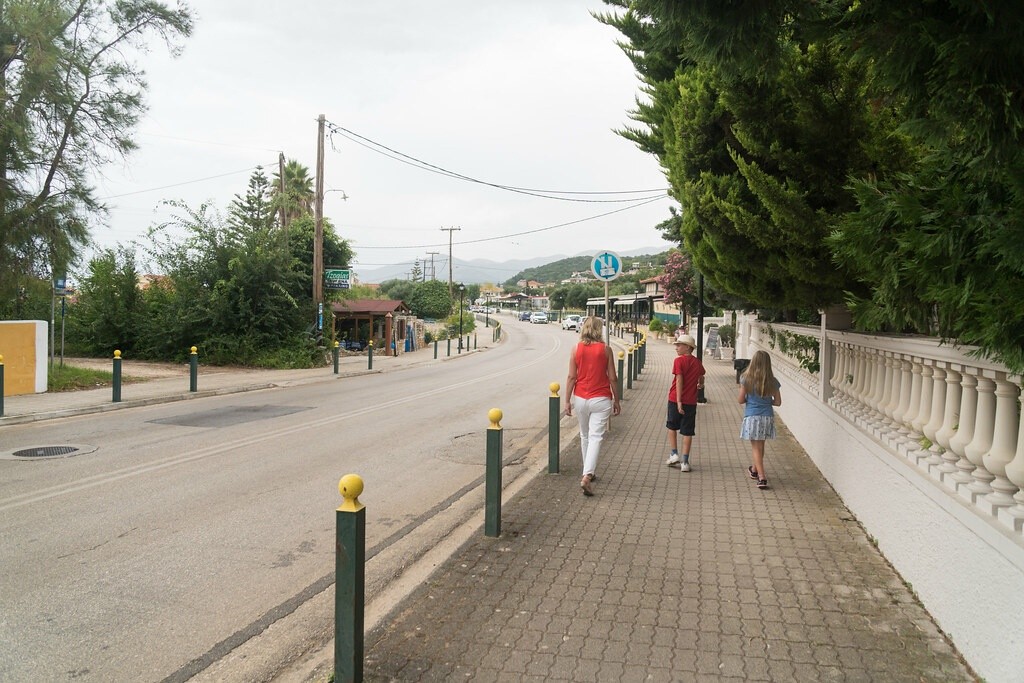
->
[749,465,758,479]
[757,479,768,487]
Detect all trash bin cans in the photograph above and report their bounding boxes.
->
[734,359,751,384]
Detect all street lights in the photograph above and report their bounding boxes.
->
[559,295,563,323]
[312,189,349,347]
[634,288,639,331]
[486,291,491,327]
[457,282,465,349]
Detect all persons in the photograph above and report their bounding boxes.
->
[565,316,620,496]
[667,335,705,472]
[615,311,620,326]
[739,350,782,487]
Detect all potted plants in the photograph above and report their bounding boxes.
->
[661,322,677,344]
[648,319,663,338]
[717,324,736,361]
[705,322,719,354]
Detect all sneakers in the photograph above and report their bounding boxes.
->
[667,454,680,465]
[681,463,691,472]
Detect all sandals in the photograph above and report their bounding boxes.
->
[585,473,597,482]
[581,477,593,496]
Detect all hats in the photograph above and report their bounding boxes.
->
[673,334,697,349]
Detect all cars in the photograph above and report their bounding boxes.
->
[339,340,366,351]
[529,312,549,324]
[518,311,532,321]
[466,305,502,314]
[562,314,582,330]
[575,316,589,333]
